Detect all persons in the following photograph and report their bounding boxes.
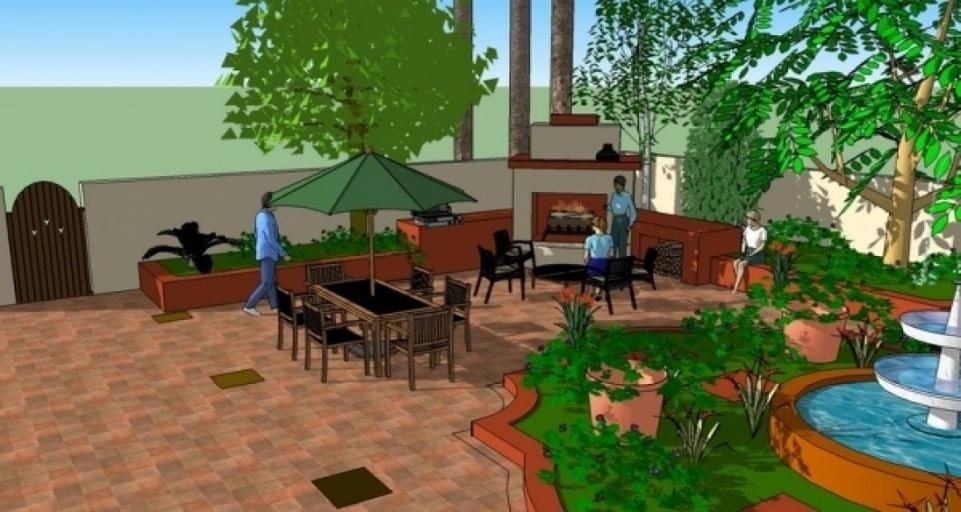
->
[730,209,769,295]
[602,174,639,258]
[242,190,293,318]
[582,215,614,302]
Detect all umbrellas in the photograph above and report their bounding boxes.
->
[268,145,478,296]
